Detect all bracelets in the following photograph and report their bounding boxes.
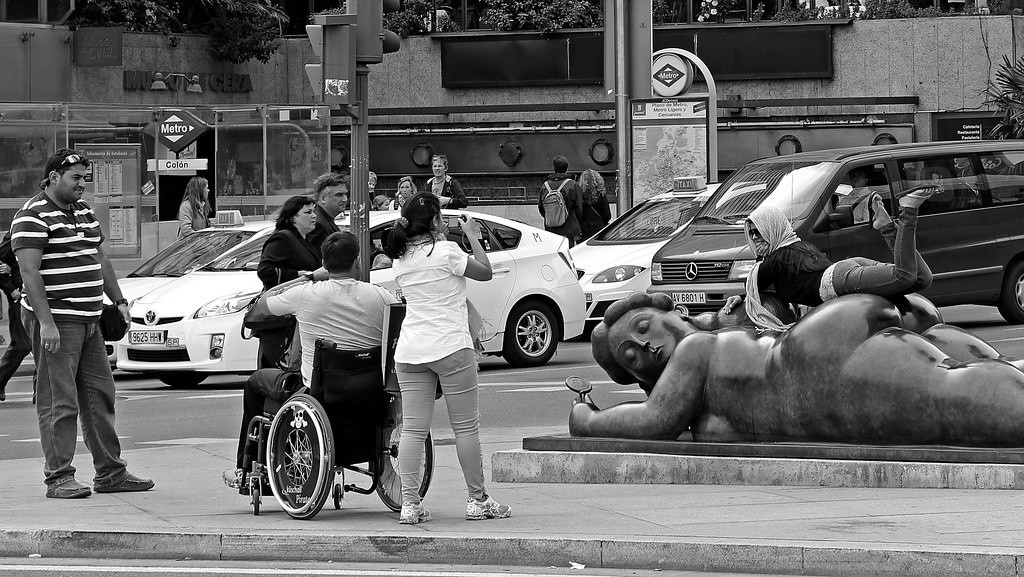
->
[114,299,128,307]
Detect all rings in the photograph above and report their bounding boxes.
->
[45,344,50,346]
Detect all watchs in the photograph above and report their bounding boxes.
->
[305,271,314,282]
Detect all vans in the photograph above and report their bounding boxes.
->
[649,140,1024,326]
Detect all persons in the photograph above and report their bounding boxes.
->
[176,176,212,239]
[388,192,512,524]
[306,173,348,264]
[10,149,155,498]
[832,166,872,225]
[538,155,583,249]
[569,292,1024,448]
[576,169,611,245]
[258,196,329,370]
[0,231,37,404]
[723,185,944,335]
[221,231,399,489]
[368,154,469,211]
[370,226,393,270]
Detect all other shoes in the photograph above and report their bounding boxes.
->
[0,388,5,401]
[93,471,155,492]
[222,468,251,486]
[46,479,91,499]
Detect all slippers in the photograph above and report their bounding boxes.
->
[867,191,883,231]
[894,184,939,199]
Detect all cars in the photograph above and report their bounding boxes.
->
[117,209,589,388]
[569,176,854,339]
[96,210,279,374]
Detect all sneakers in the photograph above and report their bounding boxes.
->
[466,494,511,520]
[398,501,432,524]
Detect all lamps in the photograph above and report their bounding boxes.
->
[149,72,203,94]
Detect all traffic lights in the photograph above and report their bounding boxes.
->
[345,0,402,64]
[303,14,357,106]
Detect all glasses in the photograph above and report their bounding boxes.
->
[748,228,762,239]
[55,152,84,170]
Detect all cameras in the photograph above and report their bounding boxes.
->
[458,215,467,227]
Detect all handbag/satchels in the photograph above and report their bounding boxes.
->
[99,302,127,341]
[242,267,296,329]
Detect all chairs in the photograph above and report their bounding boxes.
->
[866,171,891,217]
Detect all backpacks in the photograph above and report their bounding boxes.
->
[542,178,572,227]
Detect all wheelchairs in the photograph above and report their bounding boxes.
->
[221,338,436,521]
